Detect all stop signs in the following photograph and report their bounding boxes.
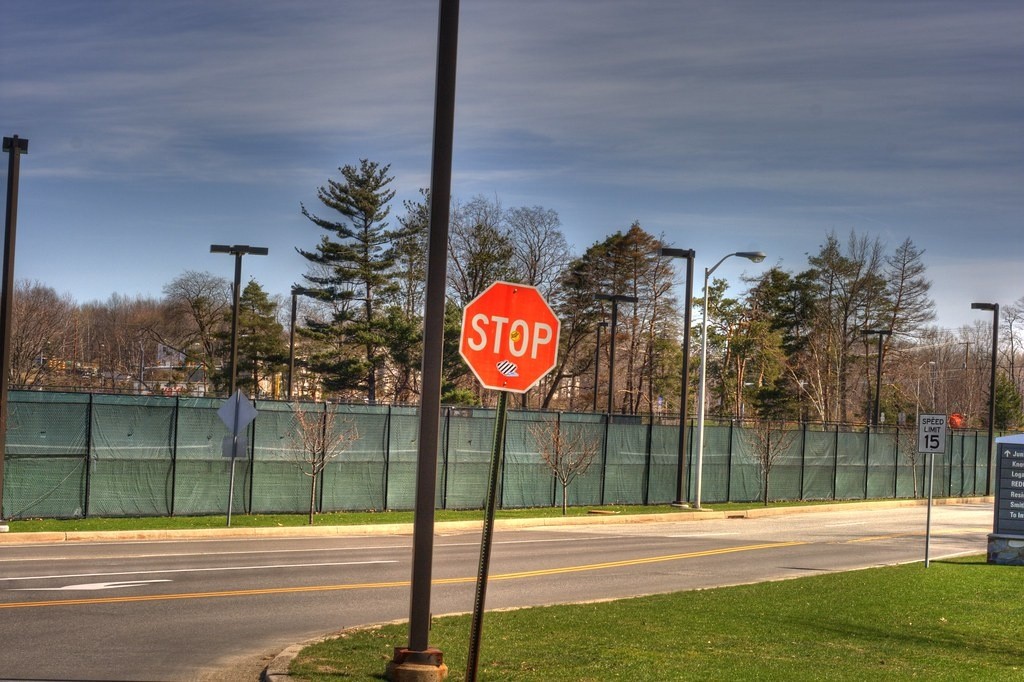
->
[459,279,561,396]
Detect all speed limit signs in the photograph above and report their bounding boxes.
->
[917,414,946,453]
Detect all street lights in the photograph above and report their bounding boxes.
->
[591,291,642,412]
[860,328,893,425]
[915,360,937,425]
[0,131,33,523]
[970,302,1000,497]
[208,244,269,392]
[284,283,310,402]
[691,250,768,510]
[661,247,699,506]
[593,321,608,412]
